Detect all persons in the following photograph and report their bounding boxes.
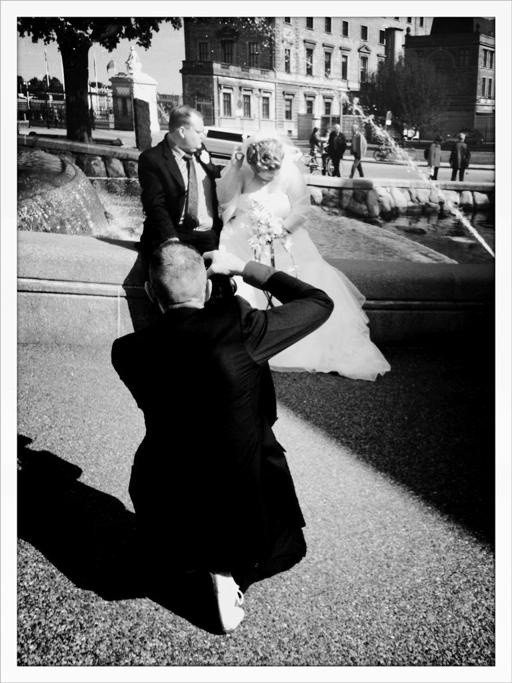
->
[138,106,223,266]
[349,122,368,178]
[321,128,332,176]
[328,122,347,177]
[212,123,395,385]
[107,243,335,637]
[424,135,443,179]
[449,131,471,182]
[308,125,327,174]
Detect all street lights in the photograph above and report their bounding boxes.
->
[23,80,32,108]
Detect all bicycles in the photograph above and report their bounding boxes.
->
[301,141,335,177]
[373,144,397,162]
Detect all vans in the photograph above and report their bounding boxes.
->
[203,126,254,157]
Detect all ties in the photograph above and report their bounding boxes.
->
[183,154,199,230]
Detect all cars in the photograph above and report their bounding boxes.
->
[444,127,484,148]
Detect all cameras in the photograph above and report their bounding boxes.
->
[204,256,237,297]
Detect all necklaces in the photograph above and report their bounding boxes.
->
[255,173,270,186]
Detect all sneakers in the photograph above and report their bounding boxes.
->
[208,572,245,633]
